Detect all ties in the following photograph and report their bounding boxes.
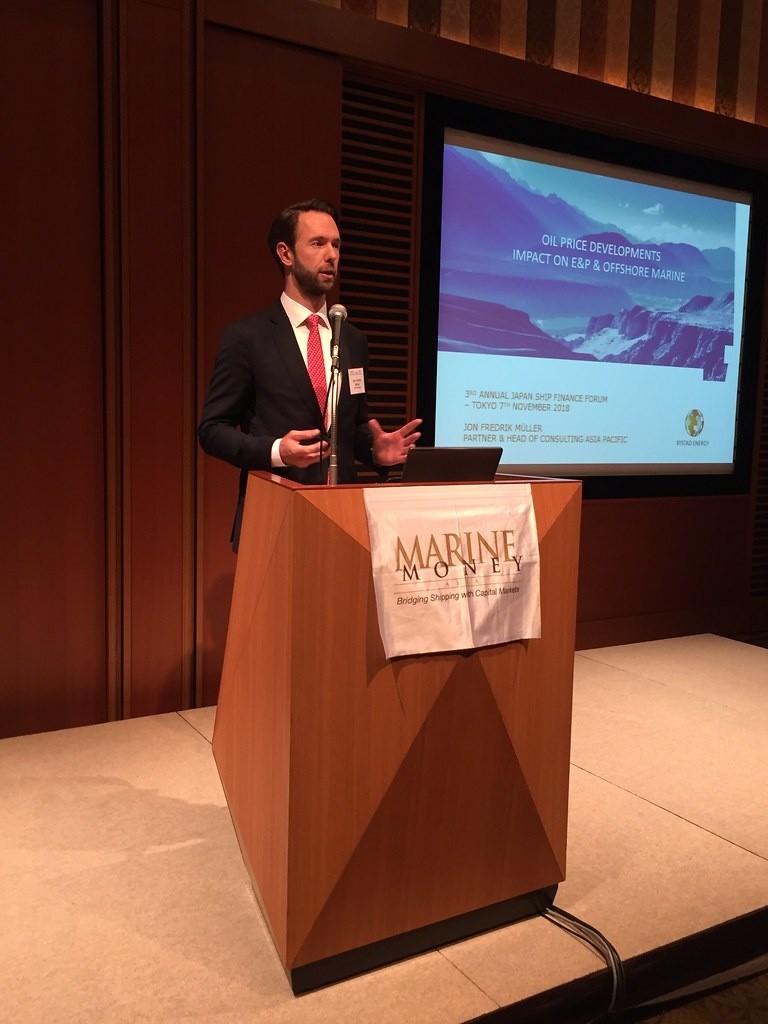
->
[306,314,329,431]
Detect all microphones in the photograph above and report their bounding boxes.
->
[329,304,348,367]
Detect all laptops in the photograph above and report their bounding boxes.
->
[400,447,503,483]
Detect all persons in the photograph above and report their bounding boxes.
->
[198,196,421,554]
